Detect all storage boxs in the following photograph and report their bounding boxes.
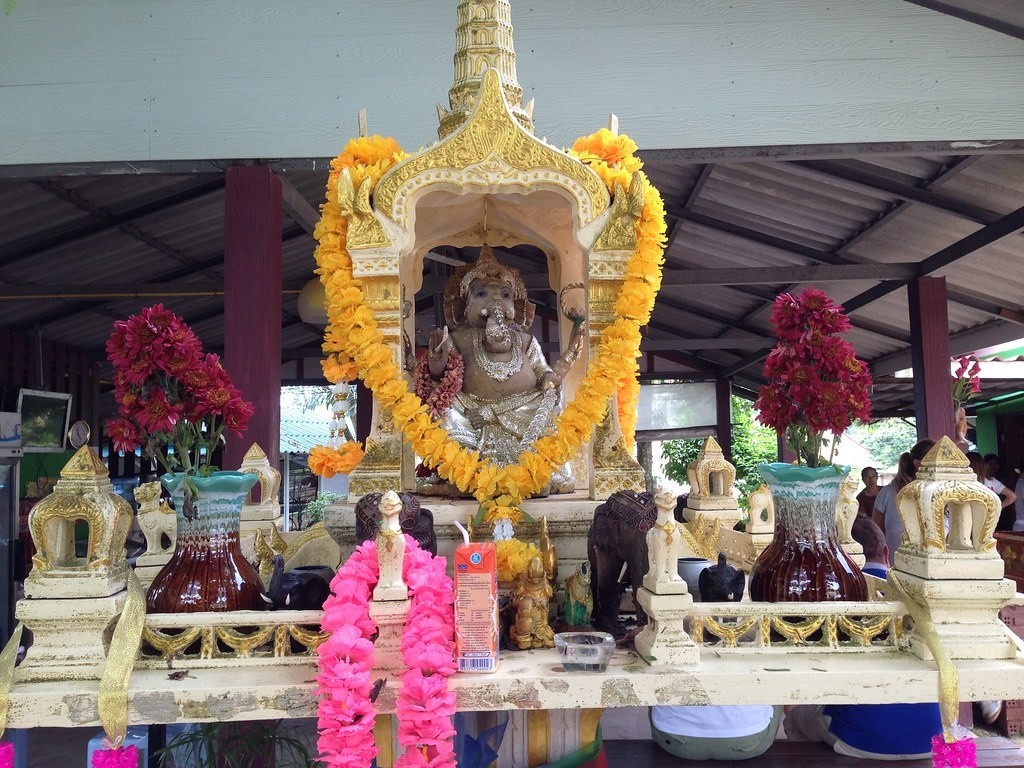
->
[456,542,500,672]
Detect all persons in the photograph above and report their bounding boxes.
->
[873,439,1002,723]
[648,493,957,761]
[965,450,1024,534]
[855,466,886,518]
[429,243,586,485]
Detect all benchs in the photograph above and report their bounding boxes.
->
[602,735,1024,768]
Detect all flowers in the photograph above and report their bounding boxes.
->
[91,745,138,768]
[951,356,983,422]
[103,301,255,521]
[930,732,978,767]
[314,537,456,768]
[308,133,669,582]
[750,285,871,474]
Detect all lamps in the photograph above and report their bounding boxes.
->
[298,275,330,325]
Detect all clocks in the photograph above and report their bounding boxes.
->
[68,420,90,450]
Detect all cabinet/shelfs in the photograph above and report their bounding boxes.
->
[1,485,1024,768]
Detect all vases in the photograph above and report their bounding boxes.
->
[748,462,869,642]
[147,469,268,654]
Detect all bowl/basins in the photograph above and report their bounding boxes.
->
[554,632,616,673]
[678,557,712,589]
